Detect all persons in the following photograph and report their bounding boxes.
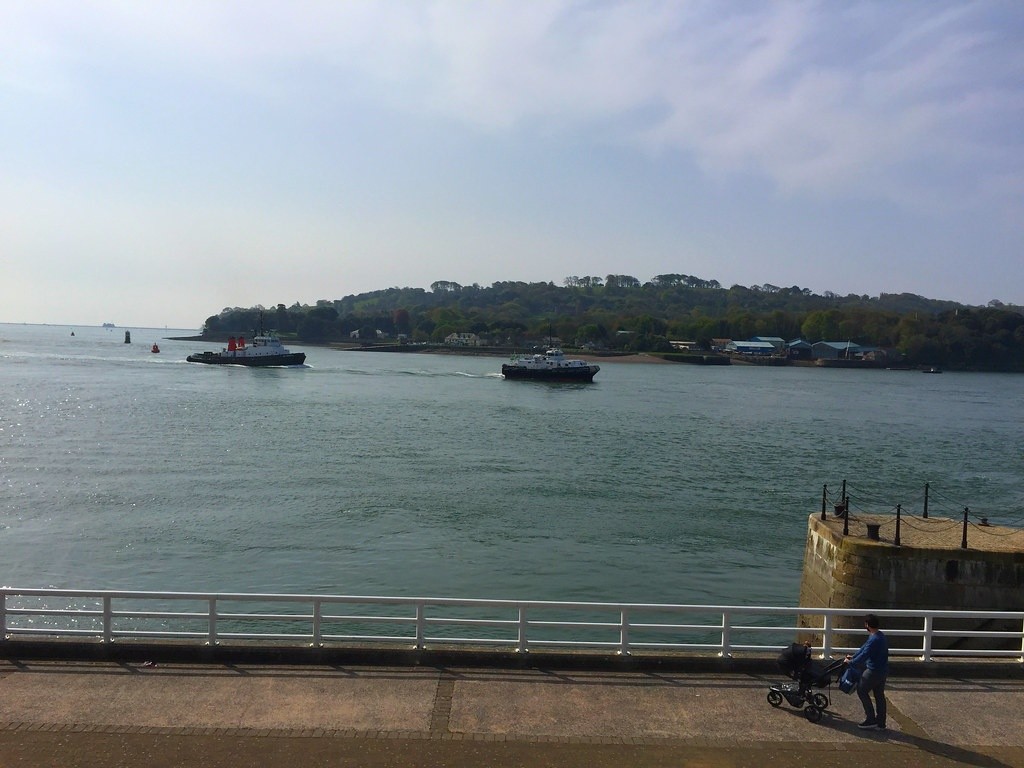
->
[844,613,889,730]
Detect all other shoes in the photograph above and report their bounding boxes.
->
[858,717,878,729]
[875,724,886,731]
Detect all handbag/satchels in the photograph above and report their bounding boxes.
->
[835,659,864,695]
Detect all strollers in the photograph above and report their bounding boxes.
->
[766,643,850,723]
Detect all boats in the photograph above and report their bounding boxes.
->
[150,342,159,353]
[501,324,601,382]
[922,367,942,374]
[185,311,308,367]
